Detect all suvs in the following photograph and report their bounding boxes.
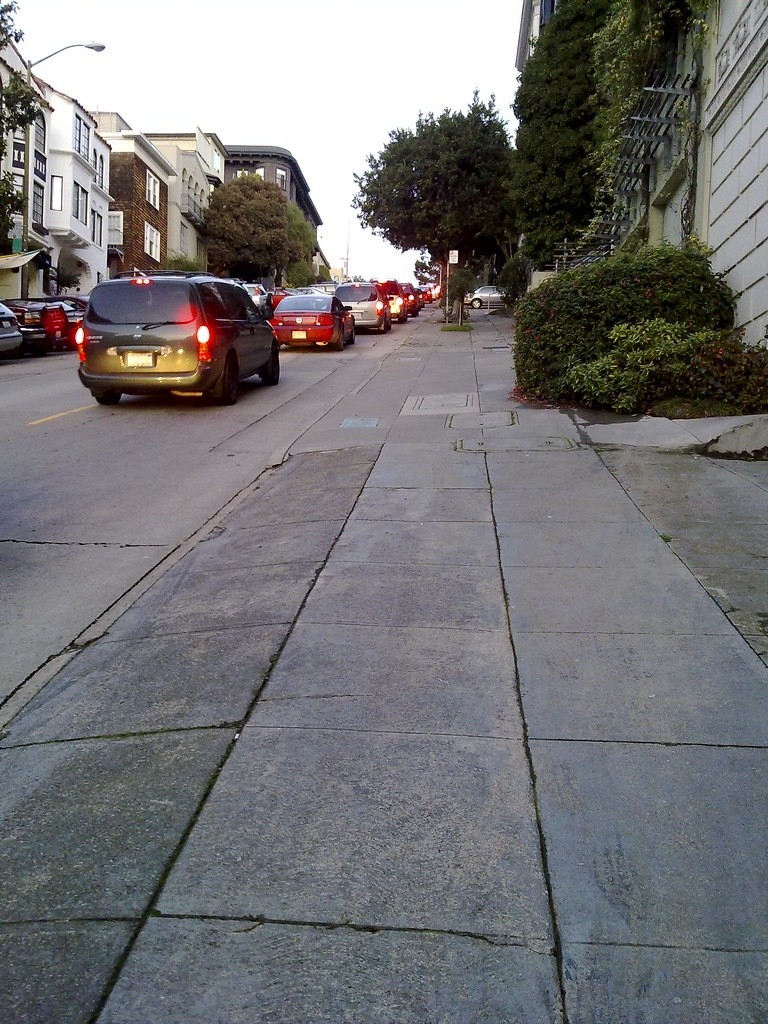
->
[369,278,412,324]
[79,269,280,406]
[336,281,395,333]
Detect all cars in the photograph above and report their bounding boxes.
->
[400,282,440,317]
[243,283,337,312]
[463,286,507,309]
[270,294,356,351]
[0,294,91,358]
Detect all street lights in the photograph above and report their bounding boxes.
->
[20,41,106,299]
